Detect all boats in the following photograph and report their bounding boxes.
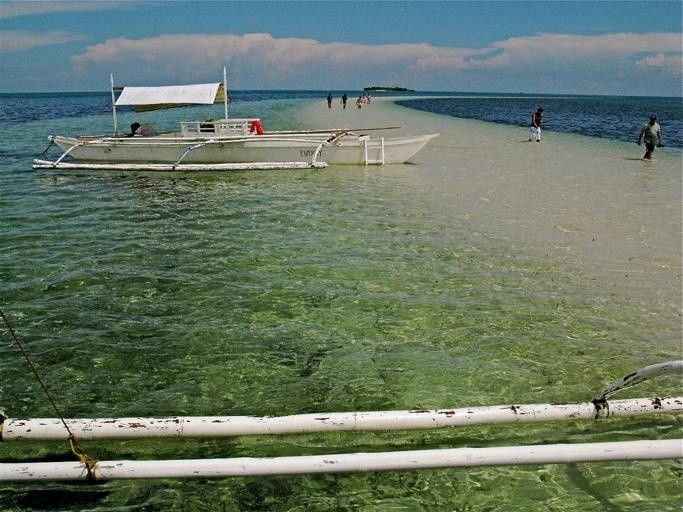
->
[29,64,444,172]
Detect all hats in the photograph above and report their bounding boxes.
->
[130,122,140,134]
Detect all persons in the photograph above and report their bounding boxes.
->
[356,92,372,110]
[340,93,348,109]
[327,93,332,108]
[638,115,662,160]
[529,108,544,142]
[124,122,157,138]
